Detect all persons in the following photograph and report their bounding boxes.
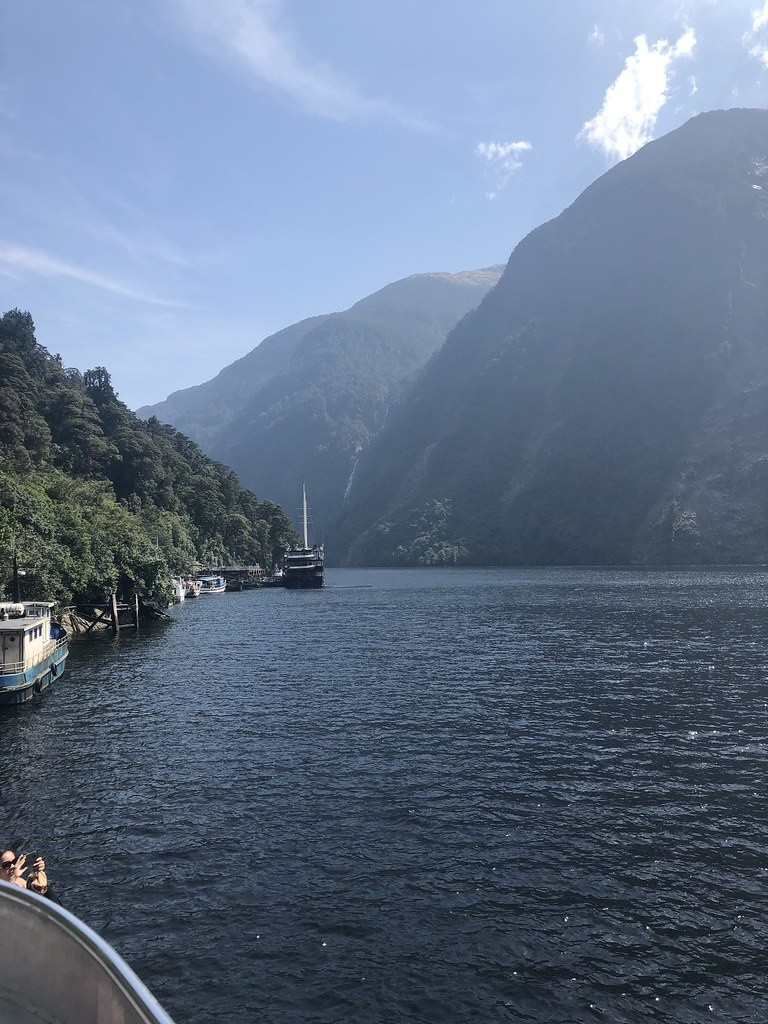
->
[0,848,44,895]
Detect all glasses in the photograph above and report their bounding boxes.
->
[1,857,19,871]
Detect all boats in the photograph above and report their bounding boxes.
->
[0,553,71,706]
[169,575,186,605]
[197,576,227,594]
[185,579,204,597]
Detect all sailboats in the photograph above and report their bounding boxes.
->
[281,481,326,589]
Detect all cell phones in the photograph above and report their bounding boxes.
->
[20,851,37,870]
